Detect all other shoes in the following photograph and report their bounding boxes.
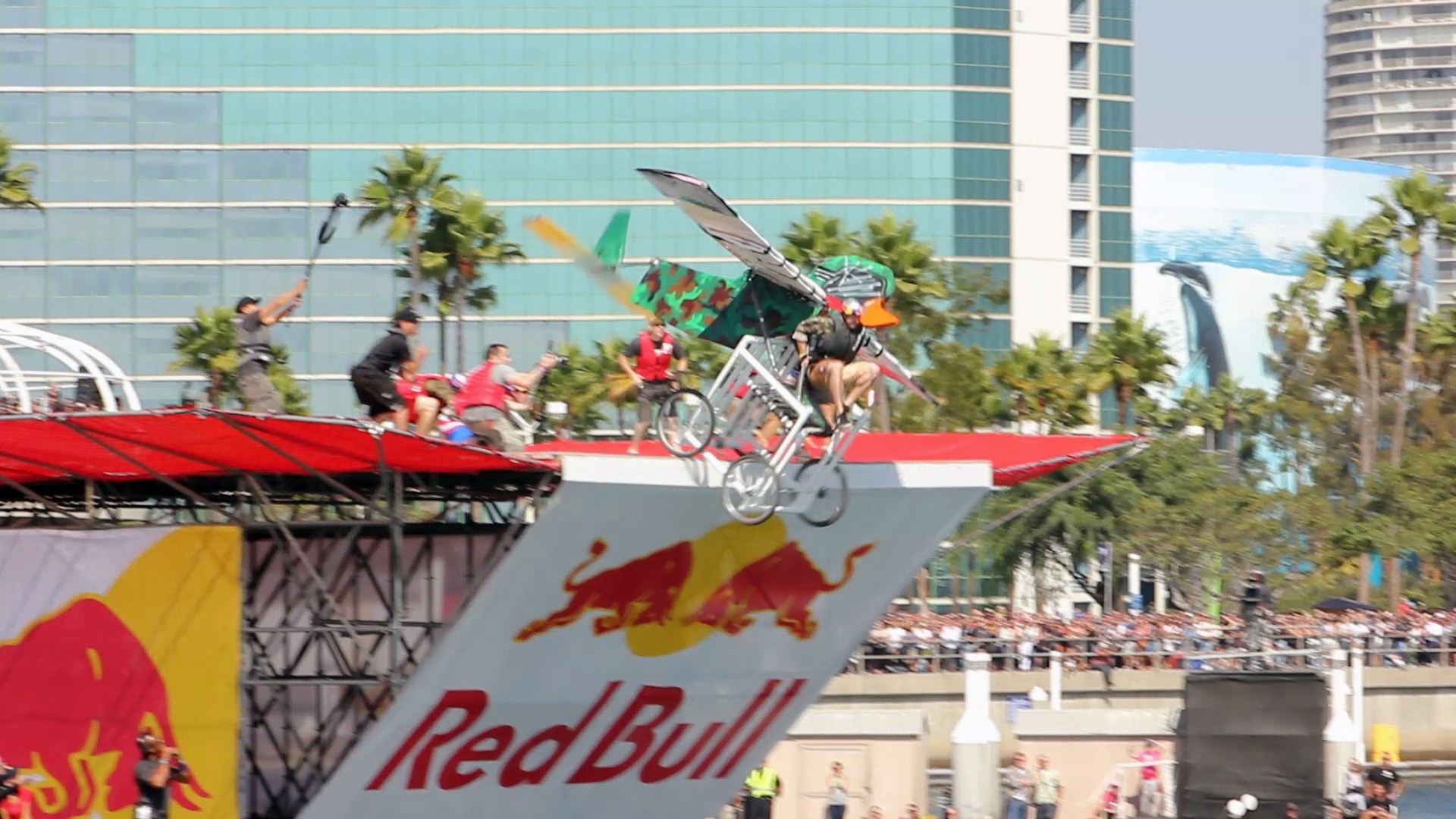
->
[628,448,637,456]
[836,413,852,428]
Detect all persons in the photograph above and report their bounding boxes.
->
[743,758,783,819]
[617,315,689,456]
[233,275,307,415]
[1001,751,1063,819]
[131,732,192,819]
[1339,750,1407,818]
[828,762,850,819]
[791,297,881,438]
[1099,737,1168,818]
[847,594,1456,672]
[458,345,558,500]
[350,306,450,439]
[906,803,922,819]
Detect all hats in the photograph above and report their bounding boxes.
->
[236,296,261,314]
[394,305,423,323]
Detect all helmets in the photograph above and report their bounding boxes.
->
[843,298,863,316]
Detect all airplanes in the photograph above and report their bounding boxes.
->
[522,166,944,528]
